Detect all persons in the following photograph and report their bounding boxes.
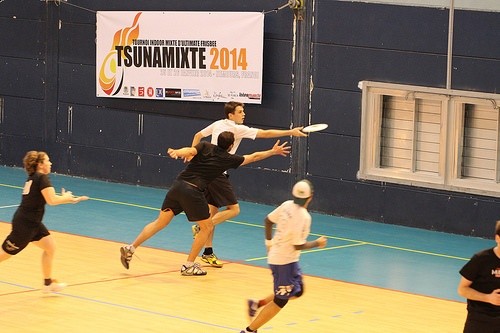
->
[181,101,308,268]
[457,220,500,333]
[120,131,291,276]
[240,177,328,333]
[0,151,90,294]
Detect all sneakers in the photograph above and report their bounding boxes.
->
[192,224,201,240]
[200,253,223,267]
[42,282,66,290]
[120,245,134,270]
[181,262,207,276]
[240,328,257,333]
[248,299,256,317]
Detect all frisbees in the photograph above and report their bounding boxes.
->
[302,123,328,132]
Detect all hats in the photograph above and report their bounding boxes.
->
[292,180,312,198]
